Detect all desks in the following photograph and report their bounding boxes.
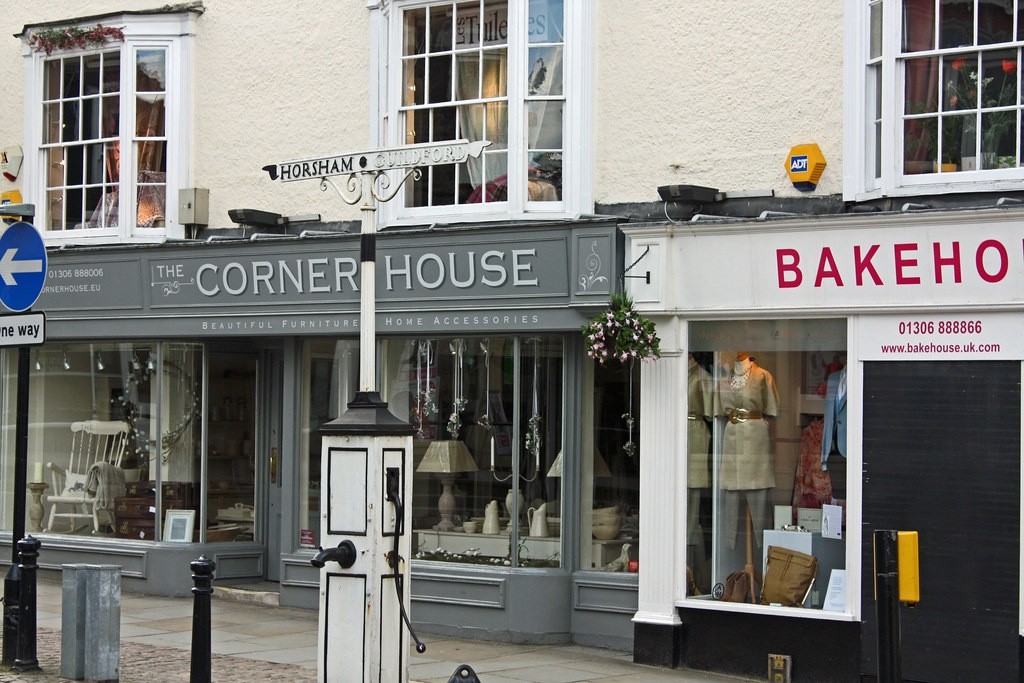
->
[215,518,254,542]
[412,528,640,572]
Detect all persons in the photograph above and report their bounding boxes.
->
[713,354,780,548]
[821,363,847,500]
[686,354,713,596]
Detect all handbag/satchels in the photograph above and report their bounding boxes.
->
[760,544,818,608]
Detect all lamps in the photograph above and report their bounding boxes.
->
[414,440,478,532]
[132,349,142,370]
[96,347,105,371]
[62,347,71,370]
[546,446,614,479]
[229,208,290,238]
[657,185,727,221]
[35,349,42,370]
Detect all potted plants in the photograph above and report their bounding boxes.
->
[909,104,961,172]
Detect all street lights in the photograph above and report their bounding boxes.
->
[0,204,36,667]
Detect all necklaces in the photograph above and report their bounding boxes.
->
[730,365,751,390]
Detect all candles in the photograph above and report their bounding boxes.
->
[535,440,541,471]
[34,463,44,483]
[489,436,495,469]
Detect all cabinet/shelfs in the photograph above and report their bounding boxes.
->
[207,420,255,499]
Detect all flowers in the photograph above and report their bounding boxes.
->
[946,58,1017,166]
[26,21,127,57]
[580,285,662,362]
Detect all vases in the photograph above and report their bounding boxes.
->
[978,130,1000,171]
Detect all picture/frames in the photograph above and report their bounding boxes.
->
[163,508,196,544]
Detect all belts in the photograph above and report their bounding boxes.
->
[727,408,766,424]
[688,416,704,421]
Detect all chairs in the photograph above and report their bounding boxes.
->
[44,419,132,538]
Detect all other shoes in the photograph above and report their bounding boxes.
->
[721,571,750,603]
